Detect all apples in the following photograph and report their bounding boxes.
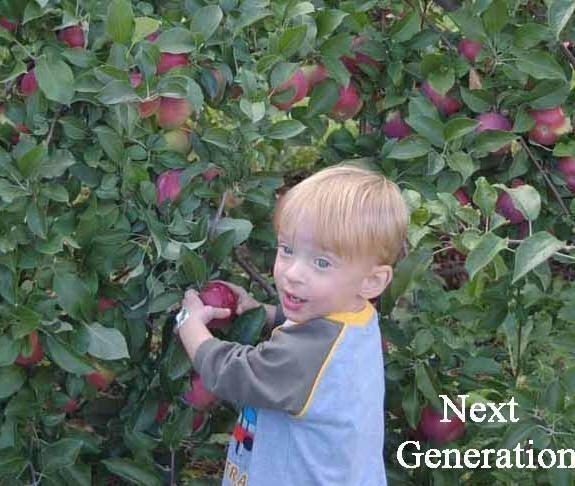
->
[0,13,575,442]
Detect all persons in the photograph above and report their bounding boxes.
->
[171,164,411,486]
